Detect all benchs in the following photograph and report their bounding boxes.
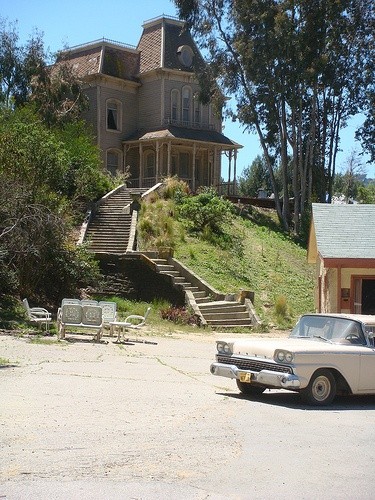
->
[61,299,117,335]
[57,305,104,342]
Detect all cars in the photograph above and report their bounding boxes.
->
[211,312,375,405]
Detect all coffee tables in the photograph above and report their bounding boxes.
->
[107,322,132,344]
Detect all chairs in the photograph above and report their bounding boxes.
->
[21,298,53,333]
[121,308,153,344]
